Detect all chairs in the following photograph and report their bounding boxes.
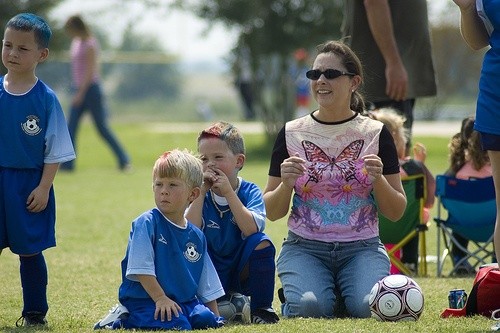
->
[379,176,430,278]
[434,172,497,278]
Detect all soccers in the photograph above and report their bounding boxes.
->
[366,272,426,322]
[213,292,251,326]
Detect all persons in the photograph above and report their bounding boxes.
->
[0,13,76,325]
[370,106,435,263]
[338,0,438,151]
[263,42,407,318]
[453,0,500,268]
[184,122,280,323]
[440,117,497,275]
[60,16,129,171]
[94,149,225,331]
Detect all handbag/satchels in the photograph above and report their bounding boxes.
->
[440,263,500,318]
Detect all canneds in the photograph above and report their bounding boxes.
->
[448,288,467,309]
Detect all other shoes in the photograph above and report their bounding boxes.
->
[16,308,49,329]
[253,307,280,326]
[404,263,416,276]
[454,258,477,274]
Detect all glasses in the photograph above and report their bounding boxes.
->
[306,69,357,80]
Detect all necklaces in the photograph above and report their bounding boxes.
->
[211,191,230,218]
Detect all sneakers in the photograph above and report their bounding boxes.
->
[94,303,130,331]
